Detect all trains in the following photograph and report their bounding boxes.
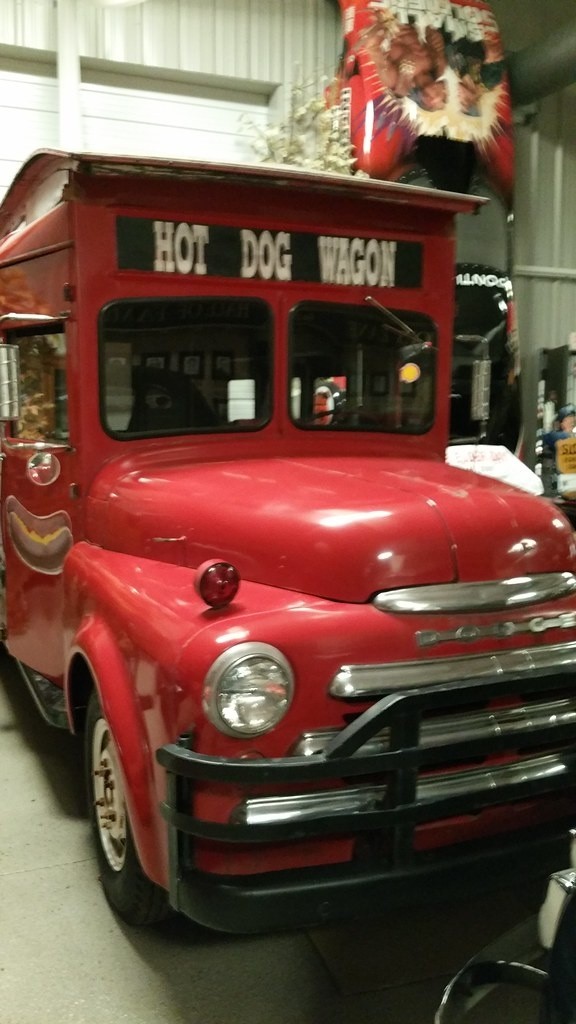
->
[0,143,576,938]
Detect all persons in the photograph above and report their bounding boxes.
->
[538,403,576,507]
[311,391,330,427]
[536,389,562,448]
